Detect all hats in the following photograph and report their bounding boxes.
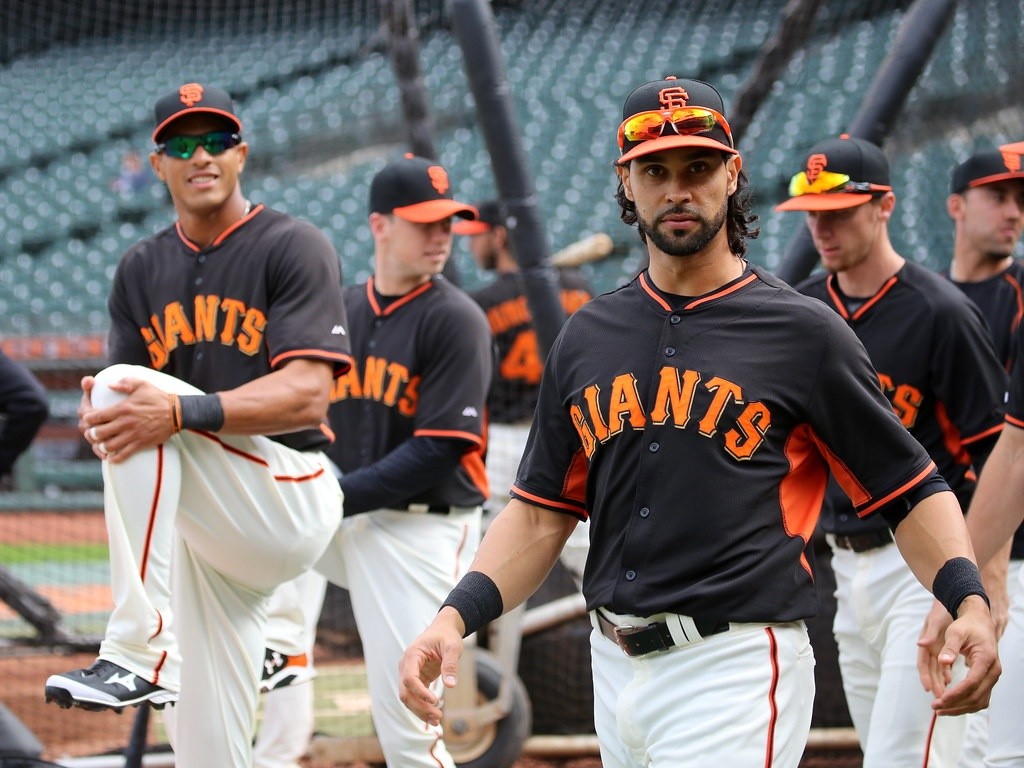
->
[617,76,739,164]
[950,141,1024,192]
[151,82,243,142]
[449,202,503,234]
[368,153,480,223]
[774,133,890,211]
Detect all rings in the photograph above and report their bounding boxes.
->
[90,427,99,440]
[98,442,109,453]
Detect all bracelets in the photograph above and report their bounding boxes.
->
[179,393,225,433]
[437,571,504,639]
[933,557,991,621]
[169,393,180,433]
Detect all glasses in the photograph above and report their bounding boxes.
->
[788,171,892,197]
[617,106,734,148]
[154,129,242,161]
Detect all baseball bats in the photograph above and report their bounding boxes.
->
[547,233,615,265]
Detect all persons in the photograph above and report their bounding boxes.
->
[257,152,494,768]
[939,150,1024,379]
[399,74,1004,767]
[917,344,1024,699]
[253,570,331,768]
[449,200,594,599]
[45,83,353,768]
[775,133,1024,767]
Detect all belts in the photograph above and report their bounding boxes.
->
[391,499,451,516]
[834,527,901,552]
[595,606,730,658]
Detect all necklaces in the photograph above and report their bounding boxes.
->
[242,200,251,218]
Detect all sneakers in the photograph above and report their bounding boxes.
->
[45,660,179,714]
[258,647,322,693]
